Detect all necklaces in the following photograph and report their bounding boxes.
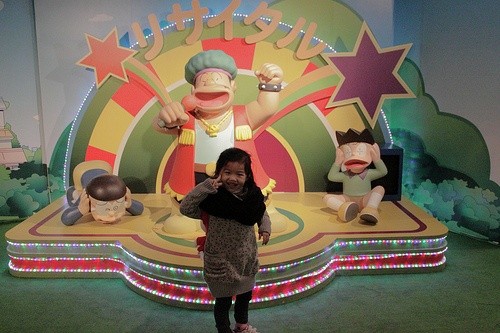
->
[194,110,233,137]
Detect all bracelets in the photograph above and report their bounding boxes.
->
[256,83,284,92]
[163,125,180,131]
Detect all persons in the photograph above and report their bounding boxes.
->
[151,50,289,233]
[61,160,144,226]
[324,128,388,223]
[179,148,271,333]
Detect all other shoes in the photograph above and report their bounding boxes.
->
[232,323,261,333]
